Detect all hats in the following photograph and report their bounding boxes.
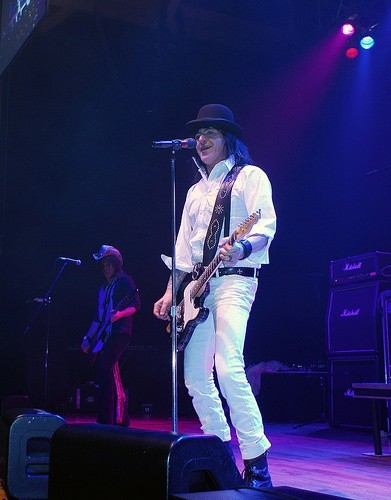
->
[93,245,123,268]
[185,104,243,140]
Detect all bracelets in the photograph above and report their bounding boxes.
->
[233,239,253,260]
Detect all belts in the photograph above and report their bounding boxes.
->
[188,266,259,279]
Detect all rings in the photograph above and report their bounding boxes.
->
[229,255,232,261]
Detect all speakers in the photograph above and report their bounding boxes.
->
[326,280,388,432]
[48,422,245,500]
[0,394,67,500]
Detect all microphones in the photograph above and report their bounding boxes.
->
[58,256,82,266]
[152,138,197,148]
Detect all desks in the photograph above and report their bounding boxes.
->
[261,370,330,423]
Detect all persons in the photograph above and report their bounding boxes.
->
[152,103,277,492]
[80,245,142,427]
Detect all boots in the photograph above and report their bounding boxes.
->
[242,450,272,490]
[222,441,236,466]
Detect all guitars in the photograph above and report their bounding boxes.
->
[89,288,141,353]
[166,208,262,352]
